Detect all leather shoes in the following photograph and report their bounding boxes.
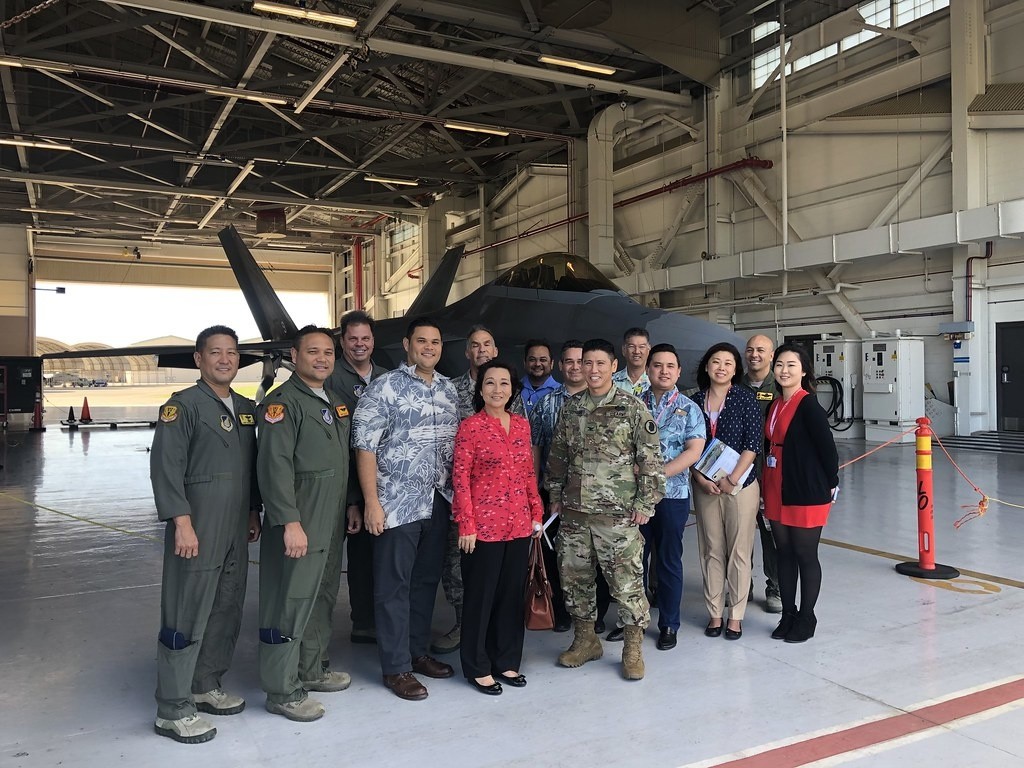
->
[469,675,503,695]
[704,618,724,637]
[383,671,428,701]
[410,655,454,678]
[494,670,526,687]
[606,627,646,642]
[657,627,677,649]
[725,619,742,640]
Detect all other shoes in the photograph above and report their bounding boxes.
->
[595,620,605,634]
[557,612,571,630]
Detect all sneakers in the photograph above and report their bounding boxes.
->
[153,713,217,744]
[193,689,246,716]
[724,588,754,607]
[351,626,378,644]
[766,595,783,613]
[302,672,350,692]
[264,696,324,721]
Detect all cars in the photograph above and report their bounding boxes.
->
[75,377,108,388]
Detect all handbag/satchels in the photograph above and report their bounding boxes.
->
[525,536,554,630]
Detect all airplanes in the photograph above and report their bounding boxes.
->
[39,224,755,404]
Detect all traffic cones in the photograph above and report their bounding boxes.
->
[65,405,78,423]
[29,388,47,432]
[78,396,93,424]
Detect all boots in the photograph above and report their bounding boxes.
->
[557,621,603,667]
[432,606,463,654]
[622,625,644,679]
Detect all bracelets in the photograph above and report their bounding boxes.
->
[727,476,738,487]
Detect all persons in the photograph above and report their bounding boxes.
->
[149,325,260,744]
[689,341,761,639]
[761,344,838,642]
[452,360,543,695]
[255,325,352,721]
[351,317,460,700]
[725,334,784,613]
[544,339,666,680]
[329,309,706,650]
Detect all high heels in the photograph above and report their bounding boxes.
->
[773,605,800,638]
[786,612,817,642]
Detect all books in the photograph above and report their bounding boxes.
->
[694,438,755,498]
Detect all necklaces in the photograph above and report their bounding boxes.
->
[527,389,541,405]
